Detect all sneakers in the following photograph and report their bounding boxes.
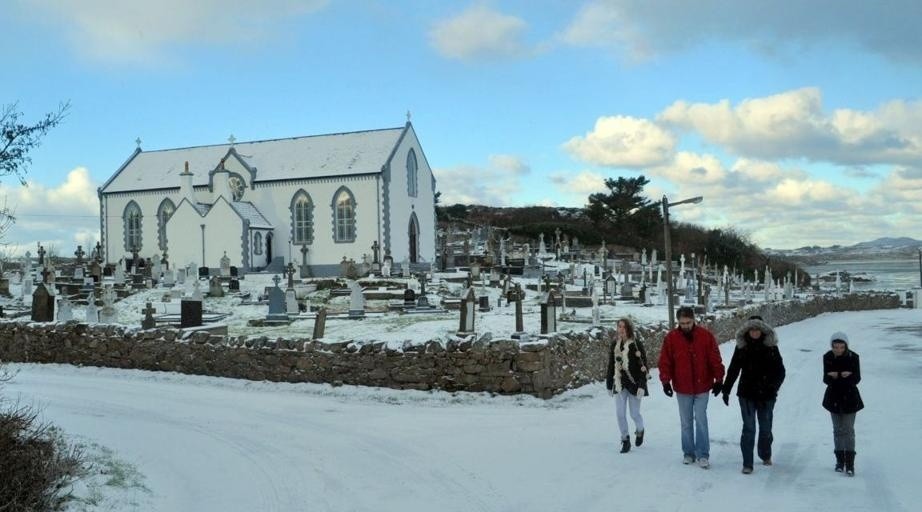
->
[695,457,709,467]
[763,457,772,467]
[635,429,643,446]
[742,462,753,474]
[683,454,695,463]
[620,435,630,454]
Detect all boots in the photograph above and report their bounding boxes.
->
[834,448,845,472]
[845,449,856,476]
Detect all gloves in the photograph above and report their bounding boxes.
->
[606,389,614,398]
[636,388,645,400]
[711,382,723,397]
[662,383,673,397]
[723,393,729,407]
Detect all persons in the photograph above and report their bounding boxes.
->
[605,316,651,454]
[719,314,786,473]
[656,307,726,469]
[818,331,866,476]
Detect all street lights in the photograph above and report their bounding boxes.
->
[661,193,703,328]
